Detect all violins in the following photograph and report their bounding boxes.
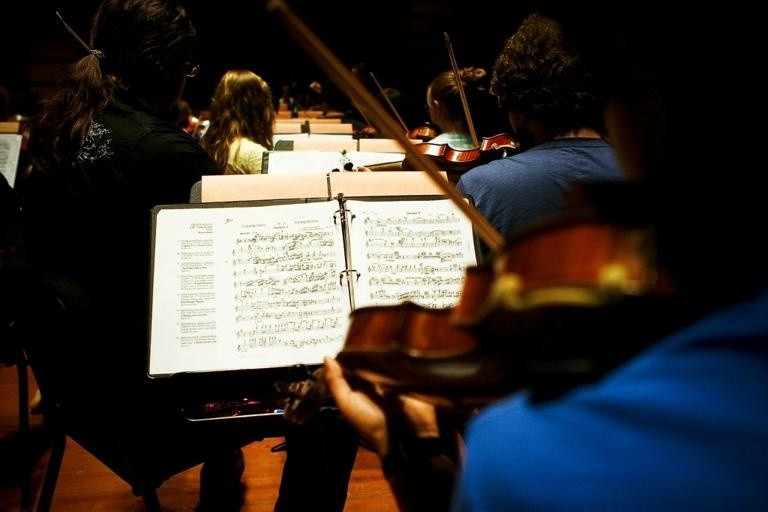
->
[358,126,436,139]
[284,225,676,429]
[332,134,520,183]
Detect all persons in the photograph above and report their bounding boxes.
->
[177,61,372,144]
[325,0,766,511]
[11,5,364,512]
[419,63,490,151]
[197,68,276,177]
[453,13,626,260]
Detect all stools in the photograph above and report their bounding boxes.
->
[32,406,277,511]
